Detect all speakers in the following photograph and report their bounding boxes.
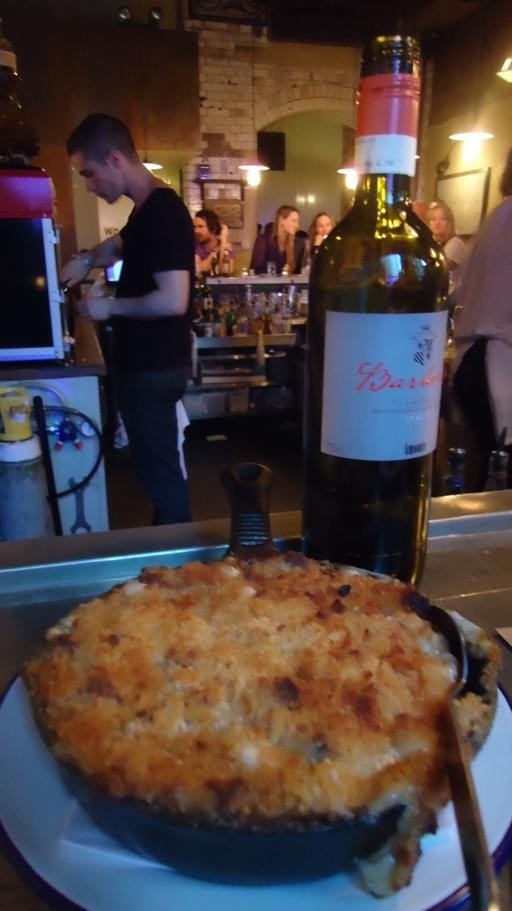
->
[257,131,285,170]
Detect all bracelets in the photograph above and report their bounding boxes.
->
[79,253,90,274]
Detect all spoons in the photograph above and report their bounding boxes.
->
[424,603,500,911]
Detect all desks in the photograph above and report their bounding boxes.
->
[203,273,311,338]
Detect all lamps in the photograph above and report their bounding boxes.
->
[238,0,271,189]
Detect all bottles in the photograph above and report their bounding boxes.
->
[441,447,468,498]
[482,450,509,492]
[300,34,453,595]
[194,236,314,337]
[256,329,266,368]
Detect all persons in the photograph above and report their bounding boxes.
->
[192,198,469,293]
[448,148,512,492]
[58,113,194,526]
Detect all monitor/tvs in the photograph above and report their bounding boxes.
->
[104,260,124,287]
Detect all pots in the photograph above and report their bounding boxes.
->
[23,461,469,889]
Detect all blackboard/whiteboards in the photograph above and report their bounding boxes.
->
[201,178,245,227]
[434,167,491,235]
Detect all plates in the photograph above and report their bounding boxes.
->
[1,665,512,911]
